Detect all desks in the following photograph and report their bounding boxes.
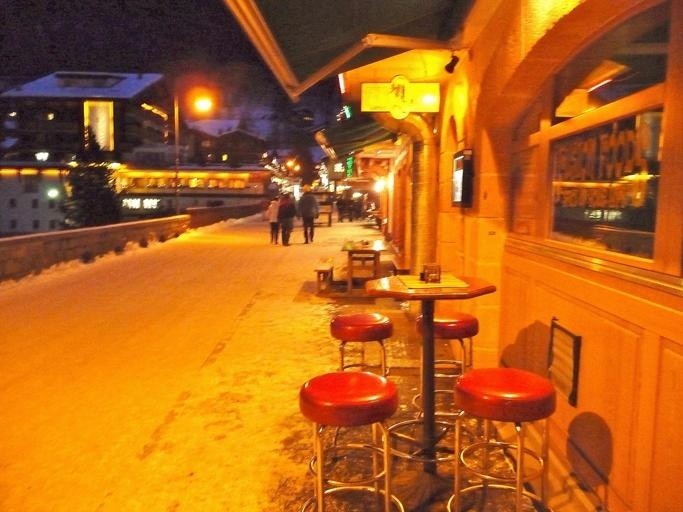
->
[364,273,498,511]
[341,240,390,299]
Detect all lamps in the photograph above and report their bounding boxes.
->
[445,50,459,74]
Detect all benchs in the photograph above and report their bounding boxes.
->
[388,257,411,276]
[314,257,336,298]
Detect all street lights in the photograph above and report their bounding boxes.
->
[175,79,213,211]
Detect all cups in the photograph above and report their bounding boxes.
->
[424,263,440,282]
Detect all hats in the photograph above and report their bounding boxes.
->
[303,185,310,193]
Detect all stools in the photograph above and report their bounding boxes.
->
[446,367,557,512]
[328,310,393,378]
[411,313,480,418]
[299,372,405,512]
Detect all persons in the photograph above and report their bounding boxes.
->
[266,192,298,246]
[336,189,376,222]
[297,185,320,244]
